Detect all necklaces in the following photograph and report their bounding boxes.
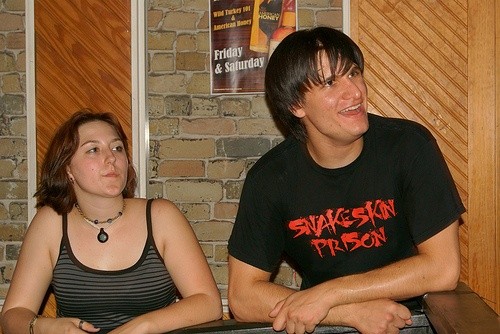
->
[73,197,128,244]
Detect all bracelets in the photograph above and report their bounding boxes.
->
[28,313,49,334]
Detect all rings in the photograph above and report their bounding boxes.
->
[77,318,84,330]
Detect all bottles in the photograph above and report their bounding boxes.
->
[268,12,296,62]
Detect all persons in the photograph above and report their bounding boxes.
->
[225,25,469,334]
[0,108,225,334]
